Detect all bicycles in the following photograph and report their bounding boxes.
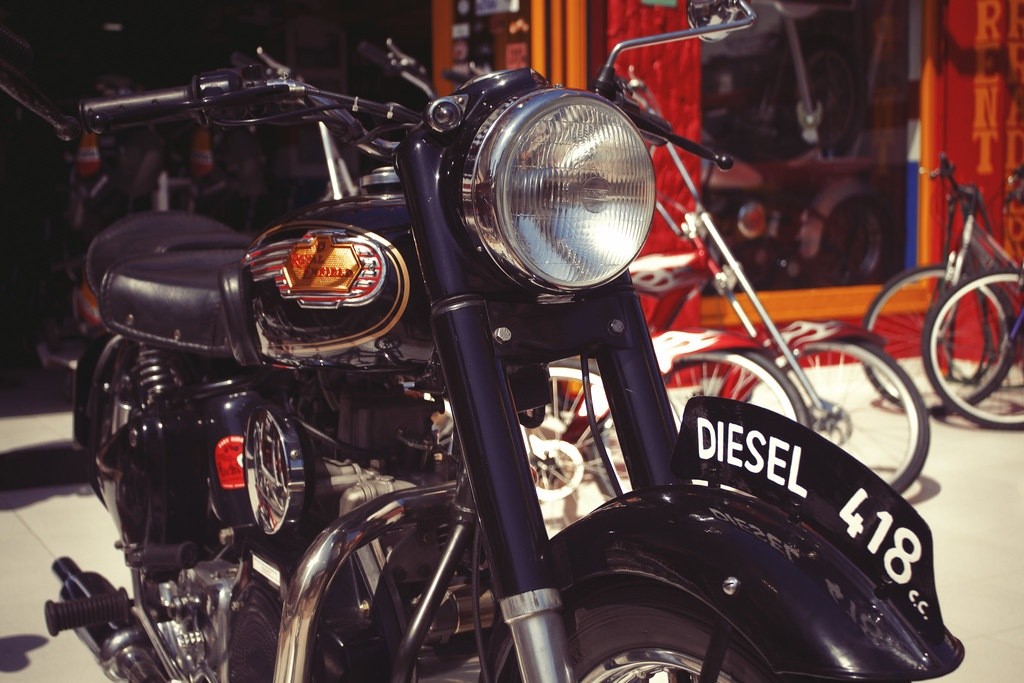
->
[859,155,1024,433]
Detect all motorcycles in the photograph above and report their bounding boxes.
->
[44,0,965,683]
